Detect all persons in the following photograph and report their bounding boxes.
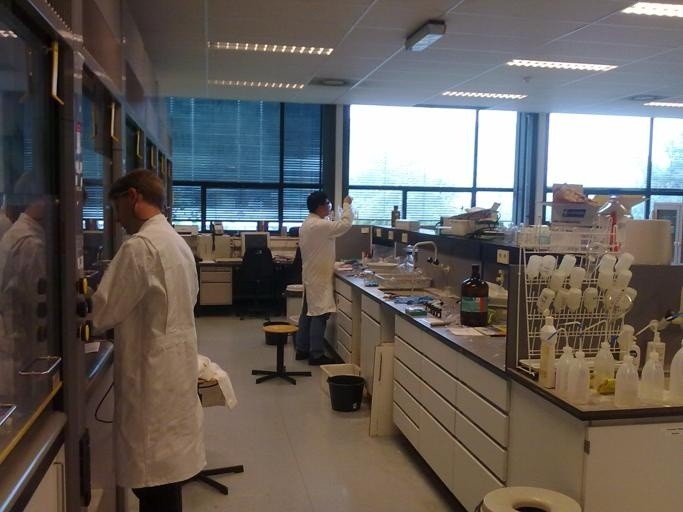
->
[295,192,352,366]
[0,166,50,399]
[0,167,30,244]
[93,171,206,511]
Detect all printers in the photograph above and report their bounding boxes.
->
[174,225,197,237]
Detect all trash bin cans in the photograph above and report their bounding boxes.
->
[286,285,305,325]
[263,321,288,345]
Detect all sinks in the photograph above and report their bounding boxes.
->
[377,286,441,298]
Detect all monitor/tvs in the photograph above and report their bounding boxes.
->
[241,232,270,257]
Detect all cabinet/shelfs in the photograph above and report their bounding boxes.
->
[360,293,396,402]
[200,265,233,306]
[329,276,361,371]
[518,215,638,377]
[392,312,510,511]
[509,378,682,512]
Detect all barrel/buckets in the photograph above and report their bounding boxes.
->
[289,315,301,351]
[286,284,304,321]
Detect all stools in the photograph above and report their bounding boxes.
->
[181,378,244,495]
[251,324,312,384]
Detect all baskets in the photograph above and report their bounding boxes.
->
[320,364,361,395]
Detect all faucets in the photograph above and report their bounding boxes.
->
[412,240,438,262]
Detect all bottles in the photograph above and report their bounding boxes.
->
[557,346,577,396]
[646,331,665,370]
[569,349,591,407]
[390,205,403,227]
[668,340,683,407]
[524,251,638,318]
[639,352,665,405]
[590,342,615,393]
[616,355,640,408]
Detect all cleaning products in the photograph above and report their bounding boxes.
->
[391,205,401,227]
